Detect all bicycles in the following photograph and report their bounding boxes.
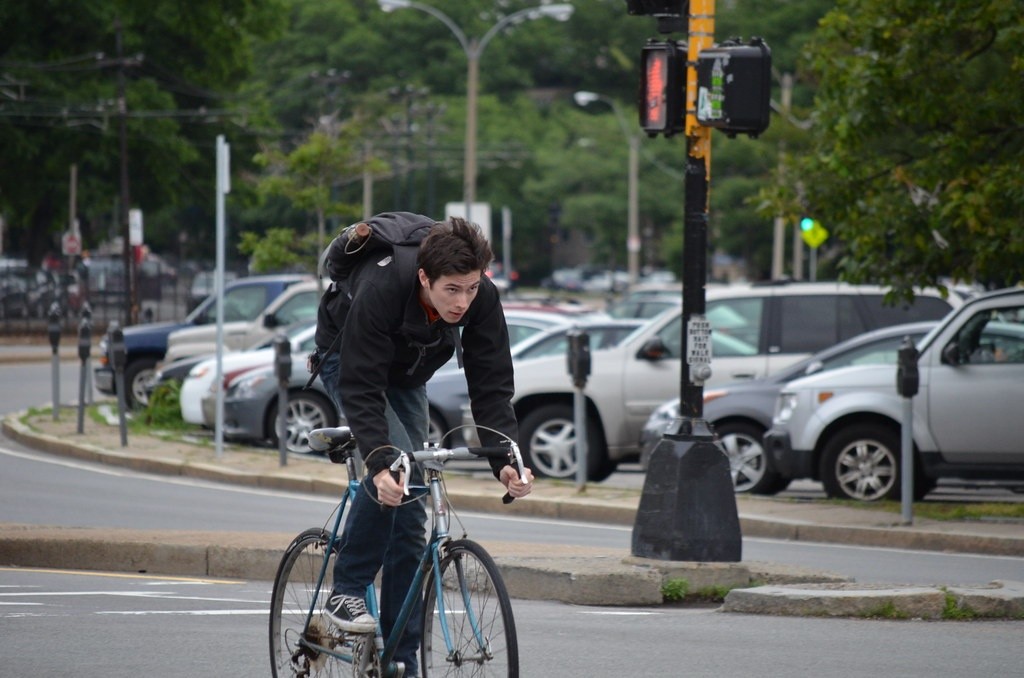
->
[268,424,539,678]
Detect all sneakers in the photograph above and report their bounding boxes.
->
[324,591,378,636]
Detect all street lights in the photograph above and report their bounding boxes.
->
[320,114,374,219]
[380,1,576,238]
[574,91,640,282]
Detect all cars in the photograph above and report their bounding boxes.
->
[91,237,182,297]
[637,318,1023,504]
[96,261,758,482]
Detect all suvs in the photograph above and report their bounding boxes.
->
[459,281,980,485]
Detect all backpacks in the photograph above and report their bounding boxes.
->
[302,207,465,392]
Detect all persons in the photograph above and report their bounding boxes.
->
[315,216,535,678]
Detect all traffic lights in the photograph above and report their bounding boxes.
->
[637,41,687,142]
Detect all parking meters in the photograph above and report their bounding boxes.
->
[895,335,921,532]
[271,335,293,467]
[566,329,592,494]
[107,319,130,445]
[75,320,91,436]
[45,303,60,423]
[77,299,95,405]
